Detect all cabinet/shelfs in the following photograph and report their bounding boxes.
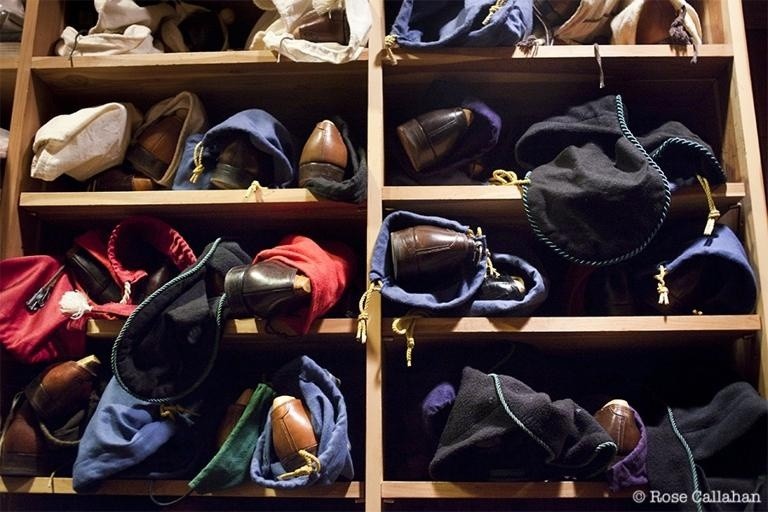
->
[0,0,27,203]
[0,0,375,512]
[376,0,768,512]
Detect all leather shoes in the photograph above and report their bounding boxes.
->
[647,267,702,315]
[92,170,156,192]
[1,401,52,477]
[637,0,683,45]
[139,264,171,305]
[215,388,255,460]
[211,134,268,190]
[68,247,122,305]
[127,109,188,183]
[297,120,346,187]
[296,8,348,44]
[391,226,477,282]
[271,397,318,473]
[536,0,577,23]
[475,272,528,302]
[594,400,639,478]
[225,260,309,317]
[24,355,103,417]
[590,269,640,315]
[396,105,471,172]
